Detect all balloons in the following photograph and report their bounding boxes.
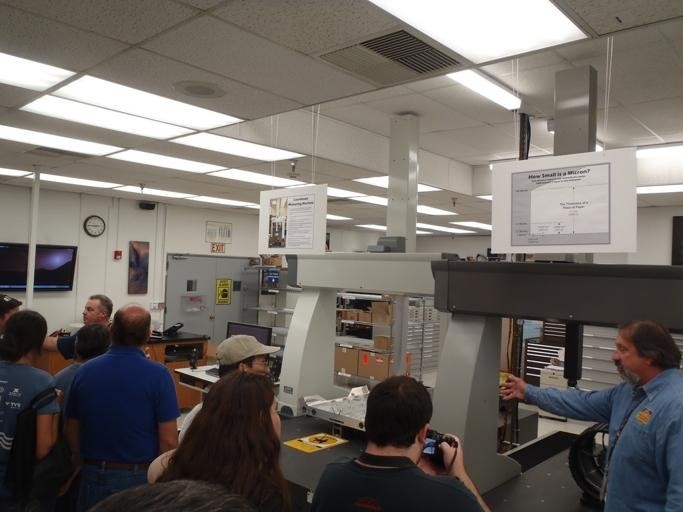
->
[83,215,107,238]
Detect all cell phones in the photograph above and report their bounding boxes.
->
[422,427,456,457]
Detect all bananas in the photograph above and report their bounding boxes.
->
[205,368,220,378]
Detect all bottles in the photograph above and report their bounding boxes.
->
[0,293,23,320]
[215,334,281,366]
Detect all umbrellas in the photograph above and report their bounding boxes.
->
[79,459,152,471]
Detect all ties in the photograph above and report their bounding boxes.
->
[0,241,78,291]
[226,321,273,360]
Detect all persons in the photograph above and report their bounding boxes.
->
[156,373,310,512]
[0,310,62,512]
[54,325,109,474]
[178,336,281,452]
[89,481,258,512]
[311,375,492,512]
[499,320,683,512]
[65,303,179,511]
[41,294,113,364]
[0,290,23,356]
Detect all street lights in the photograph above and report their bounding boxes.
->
[10,386,58,467]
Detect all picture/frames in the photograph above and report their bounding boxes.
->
[174,364,220,411]
[49,329,211,409]
